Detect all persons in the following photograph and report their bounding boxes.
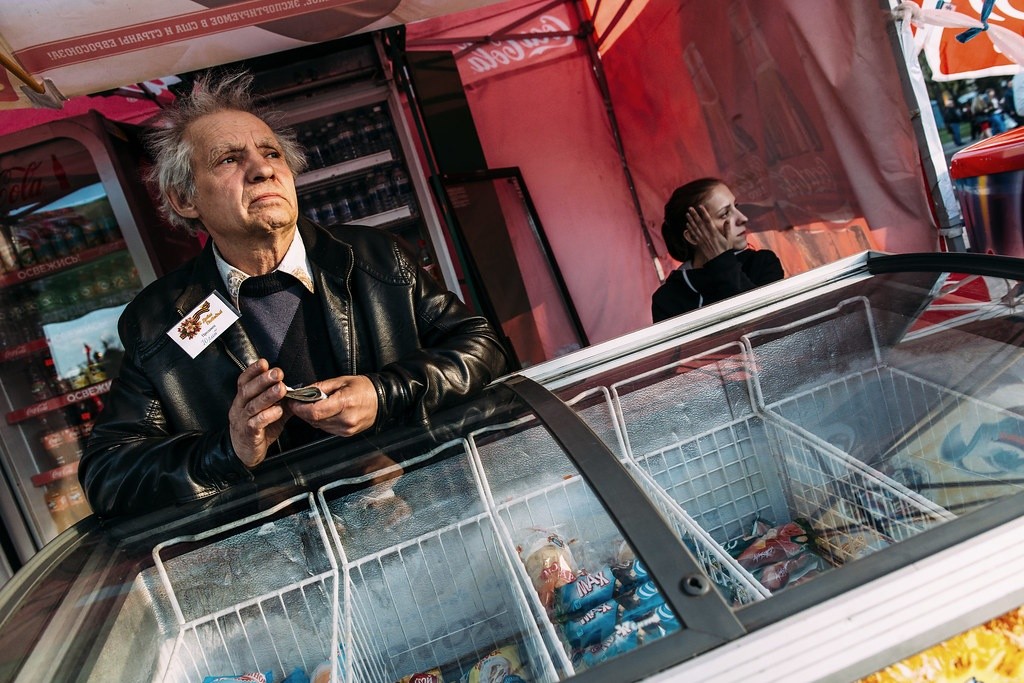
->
[651,177,784,324]
[138,393,496,636]
[77,69,509,519]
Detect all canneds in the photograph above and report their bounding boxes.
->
[0,199,120,279]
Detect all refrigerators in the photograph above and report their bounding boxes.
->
[0,252,1024,683]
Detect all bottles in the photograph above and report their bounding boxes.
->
[32,397,121,469]
[43,476,94,536]
[0,212,123,272]
[288,107,400,174]
[18,349,113,402]
[417,239,440,287]
[301,166,419,226]
[0,250,143,352]
